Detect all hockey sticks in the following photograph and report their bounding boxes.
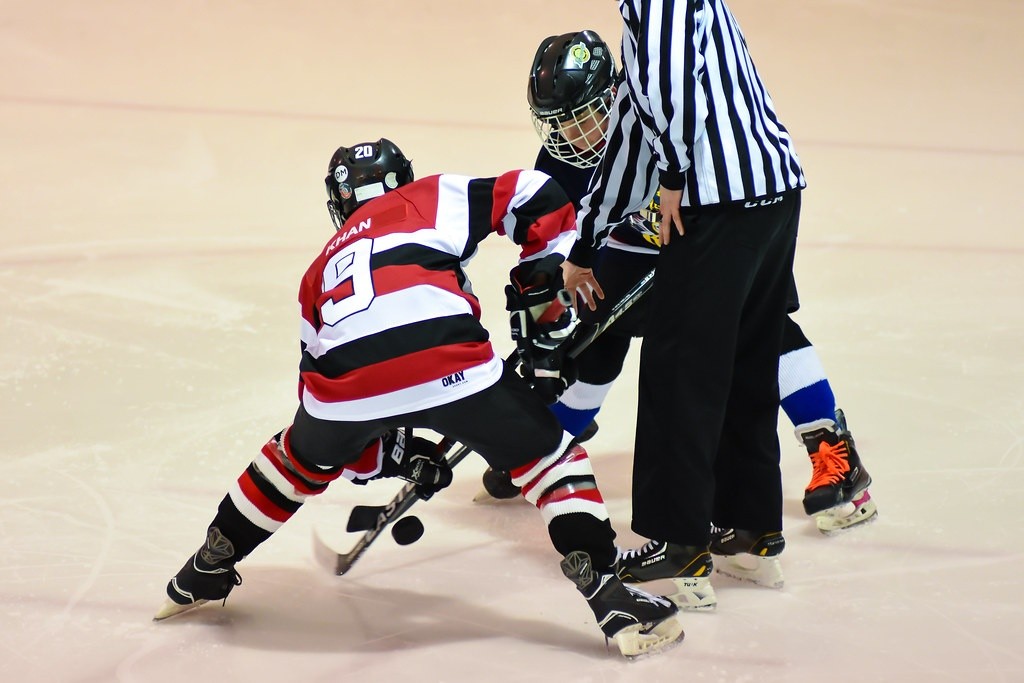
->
[309,290,573,574]
[345,267,657,534]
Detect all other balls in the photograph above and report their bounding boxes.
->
[392,515,424,545]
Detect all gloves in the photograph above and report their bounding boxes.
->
[377,429,453,501]
[504,264,577,363]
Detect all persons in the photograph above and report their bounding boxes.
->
[471,0,879,611]
[154,136,685,661]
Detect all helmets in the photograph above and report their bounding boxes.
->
[526,30,620,124]
[325,137,414,232]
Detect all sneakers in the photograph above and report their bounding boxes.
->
[474,414,599,502]
[614,534,716,612]
[560,550,685,662]
[153,546,244,620]
[793,409,878,532]
[703,525,784,589]
